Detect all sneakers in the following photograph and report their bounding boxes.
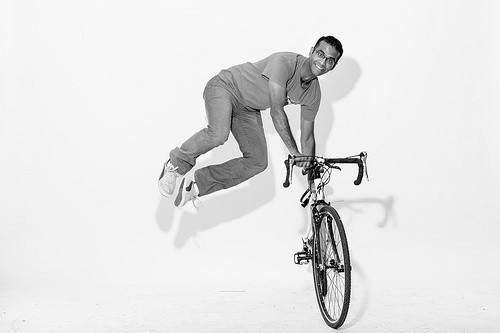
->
[174,177,197,208]
[157,156,179,197]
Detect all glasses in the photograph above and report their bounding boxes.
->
[314,48,336,65]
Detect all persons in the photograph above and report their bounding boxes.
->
[158,35,343,210]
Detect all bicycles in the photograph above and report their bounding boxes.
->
[283,150,369,330]
